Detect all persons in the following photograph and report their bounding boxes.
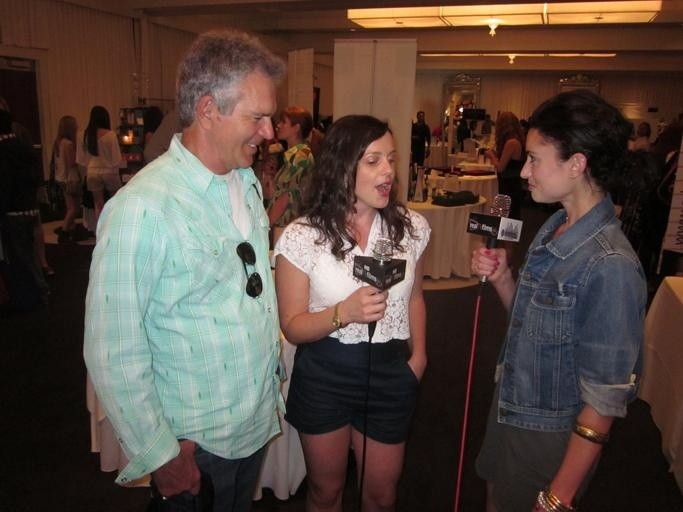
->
[77,106,122,220]
[82,28,288,511]
[143,106,164,164]
[50,116,81,230]
[1,97,56,312]
[272,115,427,511]
[624,116,651,152]
[471,88,648,512]
[255,104,333,251]
[648,116,681,277]
[414,110,461,167]
[485,111,525,219]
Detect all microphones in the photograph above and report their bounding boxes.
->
[466,194,523,297]
[353,237,408,335]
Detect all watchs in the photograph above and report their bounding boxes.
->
[332,302,343,329]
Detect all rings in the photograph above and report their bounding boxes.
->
[160,495,168,501]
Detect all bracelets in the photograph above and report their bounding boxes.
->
[536,486,573,512]
[572,422,610,444]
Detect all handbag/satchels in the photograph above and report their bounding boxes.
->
[46,178,63,203]
[80,175,95,209]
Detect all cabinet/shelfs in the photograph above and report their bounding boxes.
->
[117,107,162,172]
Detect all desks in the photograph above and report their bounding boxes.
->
[637,276,683,496]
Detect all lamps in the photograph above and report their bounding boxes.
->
[346,0,663,36]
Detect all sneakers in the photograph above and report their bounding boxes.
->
[58,228,88,246]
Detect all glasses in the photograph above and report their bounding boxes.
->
[236,242,262,298]
